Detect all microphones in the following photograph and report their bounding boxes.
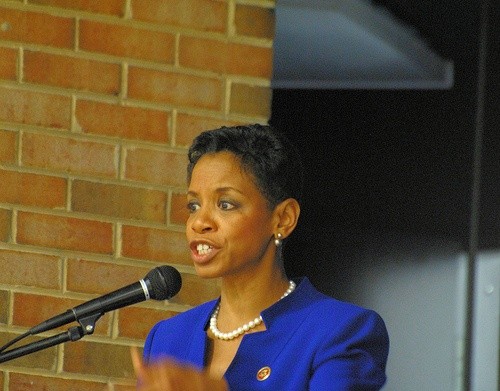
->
[28,265,183,335]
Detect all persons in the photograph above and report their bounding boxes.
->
[120,122,390,390]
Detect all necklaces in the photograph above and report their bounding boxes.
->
[208,280,296,341]
